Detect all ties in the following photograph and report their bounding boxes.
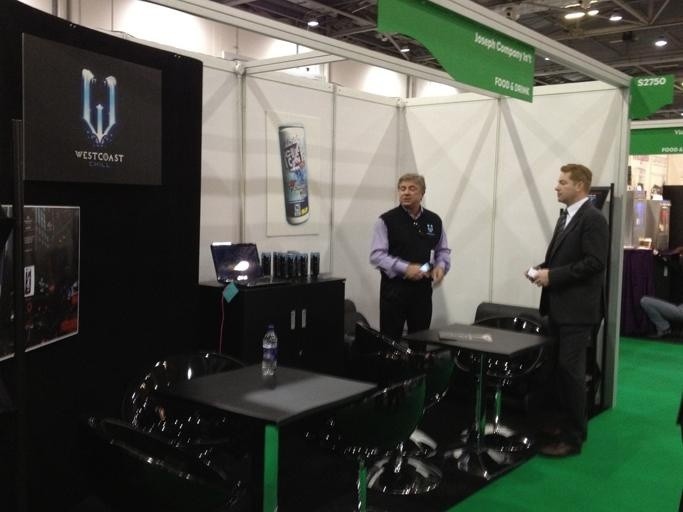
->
[548,208,568,265]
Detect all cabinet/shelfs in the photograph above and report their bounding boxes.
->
[199,274,346,379]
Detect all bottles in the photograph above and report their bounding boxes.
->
[261,325,278,376]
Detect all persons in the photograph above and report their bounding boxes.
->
[530,164,609,457]
[640,247,683,339]
[369,173,451,351]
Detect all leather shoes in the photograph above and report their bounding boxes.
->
[539,425,584,456]
[647,327,672,338]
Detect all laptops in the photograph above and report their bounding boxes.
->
[211,244,292,289]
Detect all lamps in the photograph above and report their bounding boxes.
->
[609,8,623,22]
[574,5,586,18]
[654,34,668,47]
[563,6,575,20]
[400,44,409,52]
[587,3,600,16]
[307,14,319,27]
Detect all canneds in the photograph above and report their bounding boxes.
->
[260,251,320,279]
[279,125,310,224]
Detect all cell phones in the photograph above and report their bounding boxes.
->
[419,261,433,272]
[526,266,539,280]
